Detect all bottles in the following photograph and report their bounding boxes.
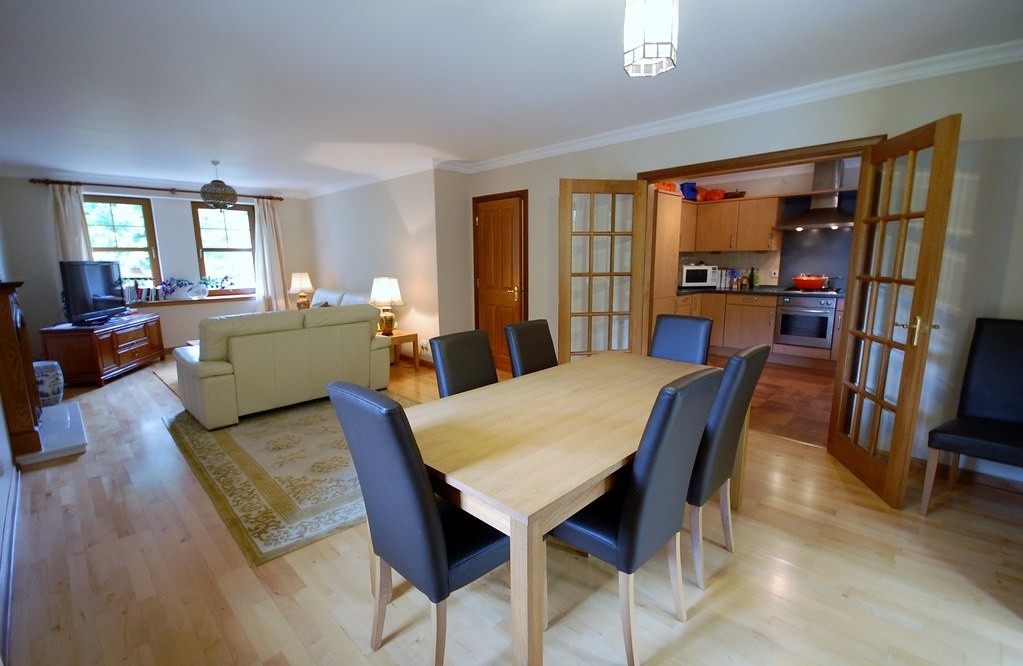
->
[731,267,760,291]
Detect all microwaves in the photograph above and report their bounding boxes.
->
[678,265,718,287]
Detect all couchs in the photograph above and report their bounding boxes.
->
[172,288,392,430]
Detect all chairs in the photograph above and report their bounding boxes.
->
[686,342,772,591]
[549,368,724,666]
[504,318,559,378]
[648,313,716,365]
[920,317,1023,515]
[328,381,548,666]
[429,329,498,399]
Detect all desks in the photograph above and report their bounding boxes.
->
[370,350,752,665]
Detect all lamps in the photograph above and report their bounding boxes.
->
[624,0,679,78]
[369,276,405,335]
[200,160,237,213]
[289,272,316,310]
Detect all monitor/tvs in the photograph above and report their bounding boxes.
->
[59,260,127,326]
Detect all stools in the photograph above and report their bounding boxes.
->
[33,361,64,407]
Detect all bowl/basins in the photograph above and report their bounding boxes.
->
[186,284,209,299]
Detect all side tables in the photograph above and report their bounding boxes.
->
[374,328,419,371]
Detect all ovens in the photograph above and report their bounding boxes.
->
[774,296,843,349]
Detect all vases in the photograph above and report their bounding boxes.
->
[185,283,207,300]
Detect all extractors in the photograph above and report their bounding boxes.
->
[772,159,854,233]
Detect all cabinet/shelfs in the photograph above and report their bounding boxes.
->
[39,313,164,387]
[653,188,845,360]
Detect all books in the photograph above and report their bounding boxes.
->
[716,269,731,289]
[137,287,165,301]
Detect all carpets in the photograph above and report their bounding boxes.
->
[153,367,423,567]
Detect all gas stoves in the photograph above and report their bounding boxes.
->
[777,287,837,295]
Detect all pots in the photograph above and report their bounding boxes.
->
[792,275,841,289]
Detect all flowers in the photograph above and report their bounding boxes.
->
[160,275,235,298]
